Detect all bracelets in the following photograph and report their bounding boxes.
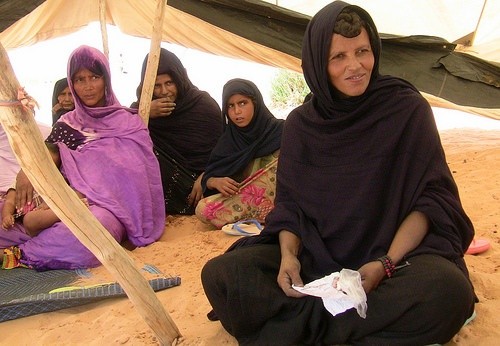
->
[375,254,397,279]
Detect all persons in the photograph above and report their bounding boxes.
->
[201,1,479,346]
[1,143,89,237]
[52,78,75,127]
[0,45,165,271]
[128,48,221,216]
[196,77,286,229]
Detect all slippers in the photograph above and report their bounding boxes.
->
[464,238,491,255]
[222,220,265,235]
